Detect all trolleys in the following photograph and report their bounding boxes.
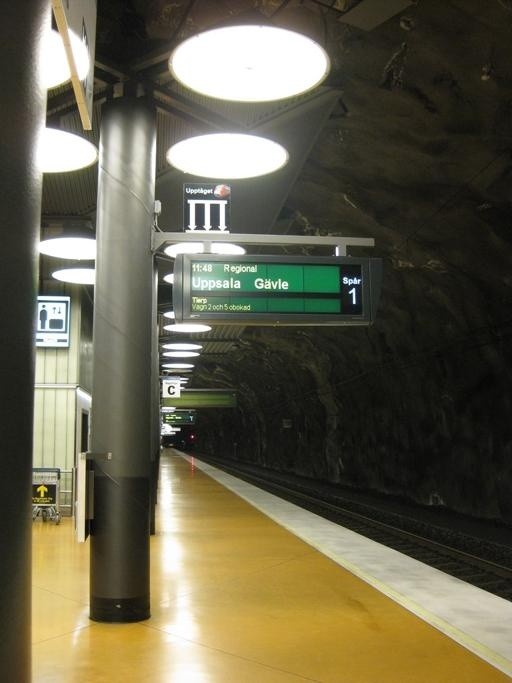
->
[32,466,64,525]
